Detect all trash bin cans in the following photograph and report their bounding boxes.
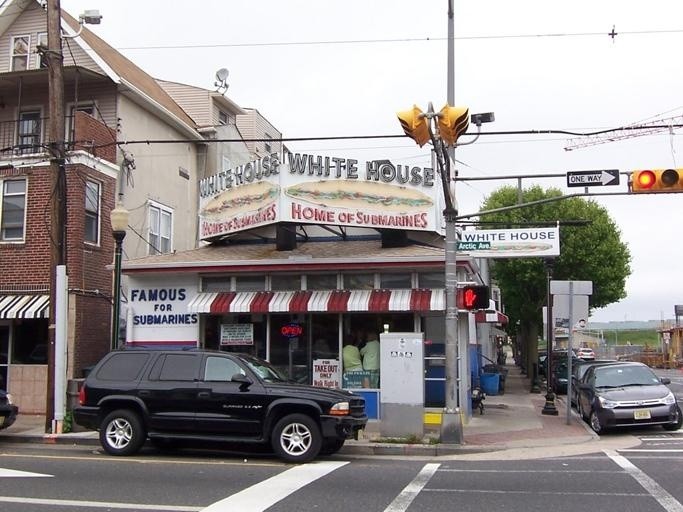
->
[66,365,97,432]
[480,363,509,396]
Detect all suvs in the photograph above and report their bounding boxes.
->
[70,347,368,462]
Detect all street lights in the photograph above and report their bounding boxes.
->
[106,193,129,349]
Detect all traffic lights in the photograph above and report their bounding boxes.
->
[438,104,470,148]
[631,168,682,194]
[462,284,490,309]
[396,104,431,149]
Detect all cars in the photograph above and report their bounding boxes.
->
[537,346,682,435]
[0,389,18,429]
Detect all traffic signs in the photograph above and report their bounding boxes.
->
[566,169,620,186]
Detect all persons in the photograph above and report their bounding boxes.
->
[360,334,379,389]
[342,334,363,371]
